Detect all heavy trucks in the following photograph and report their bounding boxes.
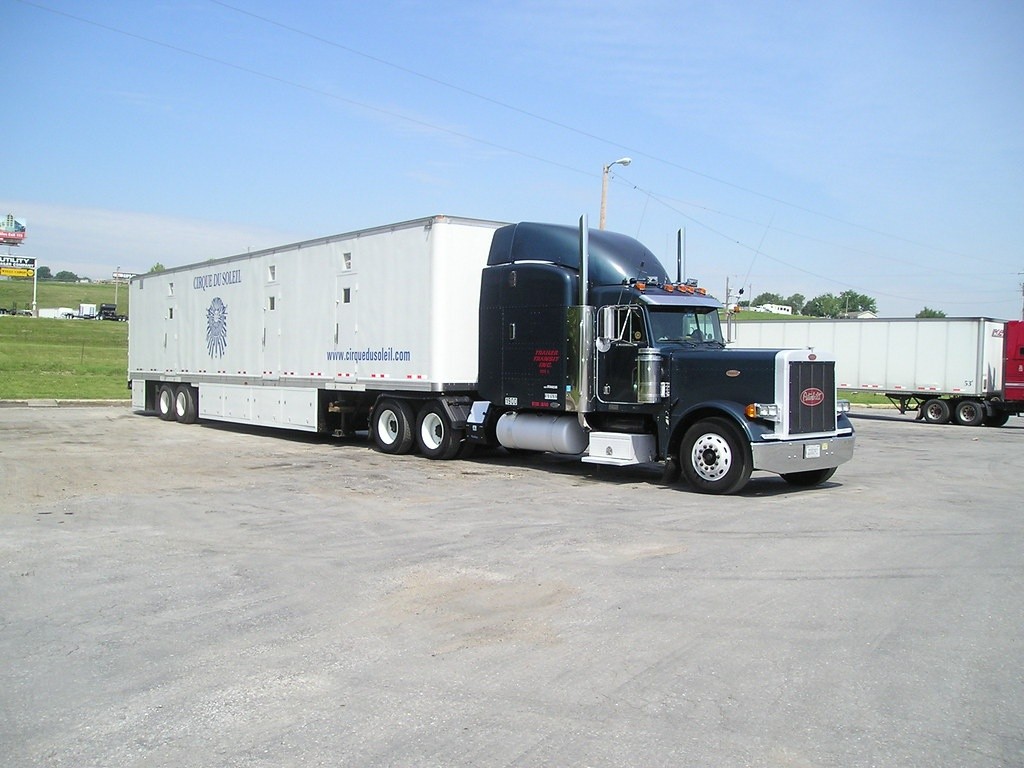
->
[686,316,1023,427]
[127,214,857,495]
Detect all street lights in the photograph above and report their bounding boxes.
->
[600,158,632,230]
[114,265,121,312]
